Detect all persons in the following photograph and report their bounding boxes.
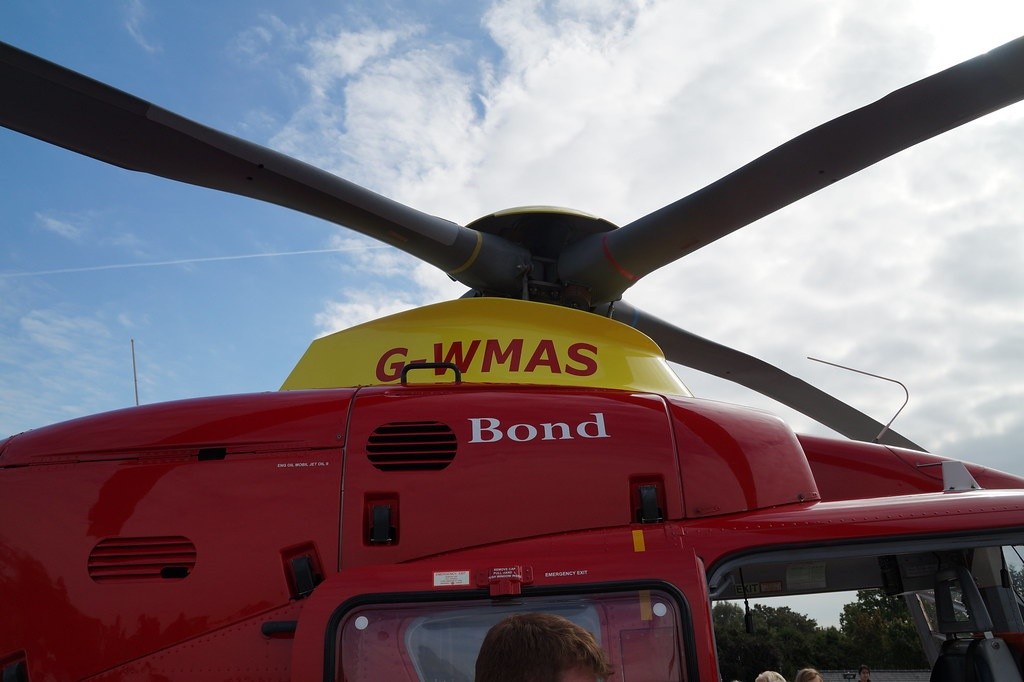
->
[474,611,616,682]
[858,664,872,682]
[755,671,787,682]
[795,668,823,682]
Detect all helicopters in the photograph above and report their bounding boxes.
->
[0,32,1023,682]
